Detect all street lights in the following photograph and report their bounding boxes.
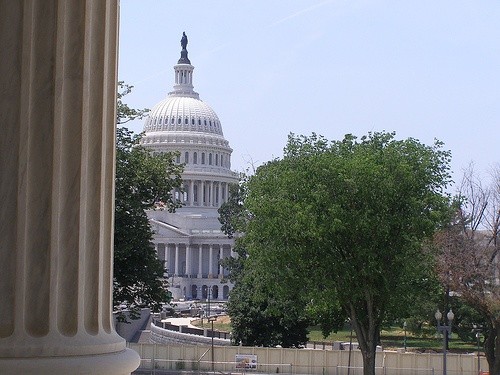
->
[471,323,484,375]
[434,307,455,375]
[204,285,213,317]
[207,313,219,371]
[404,322,408,351]
